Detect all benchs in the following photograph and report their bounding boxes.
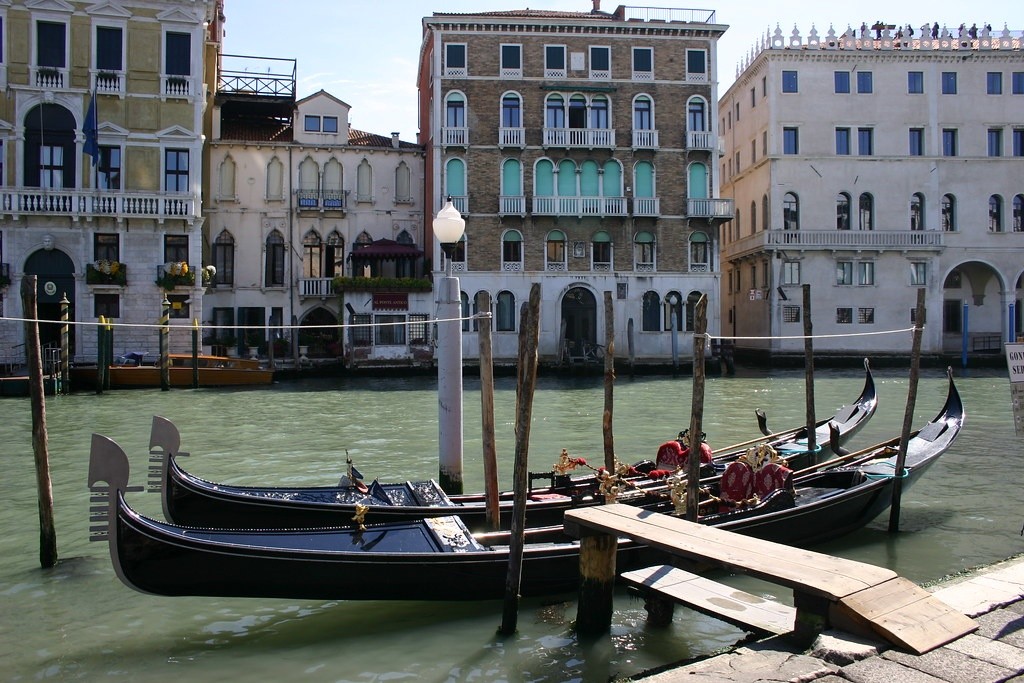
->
[656,440,799,515]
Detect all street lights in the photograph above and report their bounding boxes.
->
[432,194,465,502]
[670,294,678,361]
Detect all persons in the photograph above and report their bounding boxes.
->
[861,21,991,39]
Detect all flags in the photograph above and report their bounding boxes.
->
[83,87,99,167]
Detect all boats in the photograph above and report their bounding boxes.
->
[68,351,275,389]
[42,365,966,603]
[149,356,879,526]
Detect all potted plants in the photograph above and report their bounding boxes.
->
[223,335,239,358]
[297,335,311,360]
[246,335,261,360]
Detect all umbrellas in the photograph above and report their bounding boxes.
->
[349,238,425,277]
